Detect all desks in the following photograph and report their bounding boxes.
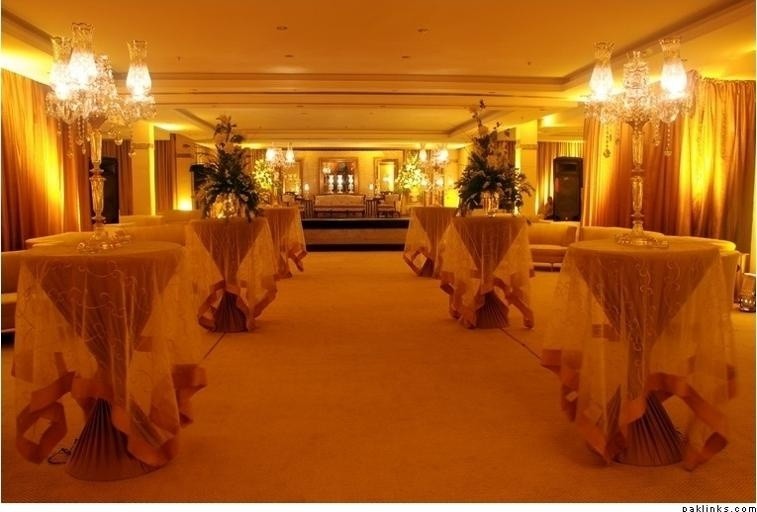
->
[7,244,207,484]
[311,195,366,216]
[401,206,457,277]
[433,217,537,330]
[540,240,716,467]
[262,208,307,281]
[193,219,280,334]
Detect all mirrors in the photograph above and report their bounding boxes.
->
[316,154,360,195]
[278,156,303,196]
[373,157,399,195]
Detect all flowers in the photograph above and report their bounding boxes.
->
[451,99,532,219]
[193,112,260,219]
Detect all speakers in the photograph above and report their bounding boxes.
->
[89,154,120,224]
[552,156,583,222]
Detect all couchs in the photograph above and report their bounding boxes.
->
[525,217,740,325]
[2,208,191,336]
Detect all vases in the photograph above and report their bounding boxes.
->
[482,192,499,219]
[212,193,238,219]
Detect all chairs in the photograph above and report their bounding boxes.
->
[280,192,305,217]
[376,191,399,218]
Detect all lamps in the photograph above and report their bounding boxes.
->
[576,35,692,248]
[260,137,301,207]
[407,138,457,205]
[337,174,344,194]
[347,173,354,194]
[326,175,334,194]
[49,21,163,252]
